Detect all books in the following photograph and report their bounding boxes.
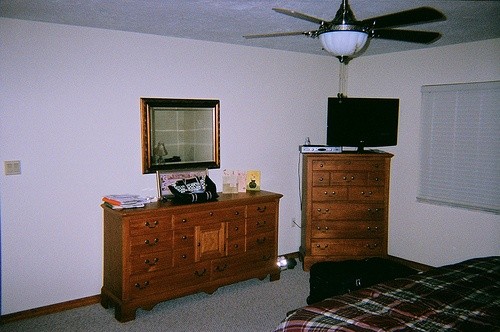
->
[102,195,144,210]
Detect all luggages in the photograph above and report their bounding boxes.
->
[306,257,418,306]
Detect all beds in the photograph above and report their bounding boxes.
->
[275,254,500,332]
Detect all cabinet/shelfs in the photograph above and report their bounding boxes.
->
[98,188,284,322]
[297,149,394,272]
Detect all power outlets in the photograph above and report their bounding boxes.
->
[292,217,296,227]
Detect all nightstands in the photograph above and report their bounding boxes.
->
[163,156,182,162]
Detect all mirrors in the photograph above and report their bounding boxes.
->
[141,97,221,175]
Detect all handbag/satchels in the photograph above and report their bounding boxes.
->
[168,174,220,205]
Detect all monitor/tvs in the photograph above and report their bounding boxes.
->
[327,97,399,154]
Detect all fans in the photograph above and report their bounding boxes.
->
[241,0,447,45]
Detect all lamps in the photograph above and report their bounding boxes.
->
[154,142,168,165]
[316,22,375,64]
